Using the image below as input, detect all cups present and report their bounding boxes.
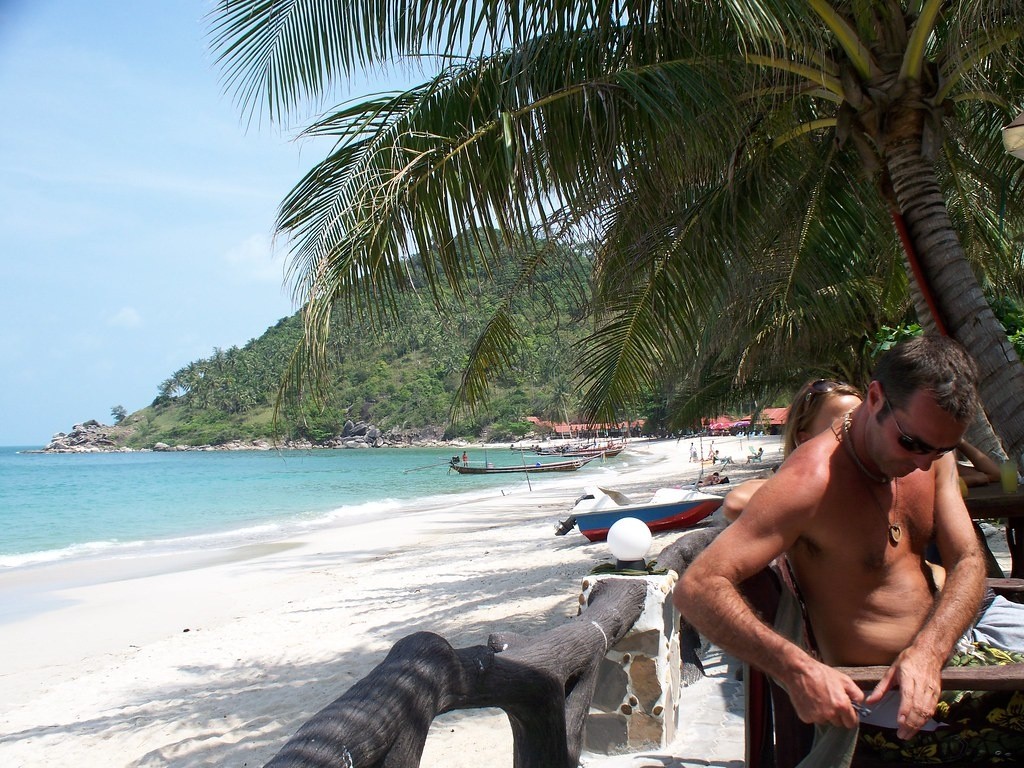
[999,460,1017,494]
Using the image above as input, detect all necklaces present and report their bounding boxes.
[842,402,892,484]
[831,413,901,543]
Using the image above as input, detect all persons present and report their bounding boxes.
[689,443,697,463]
[713,450,736,465]
[705,440,716,461]
[746,448,762,463]
[691,472,722,487]
[462,451,468,467]
[673,336,1024,768]
[531,439,615,453]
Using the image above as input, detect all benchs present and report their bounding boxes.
[743,554,1024,768]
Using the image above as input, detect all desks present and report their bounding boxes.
[964,481,1024,580]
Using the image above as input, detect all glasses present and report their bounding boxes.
[882,392,958,457]
[807,380,849,410]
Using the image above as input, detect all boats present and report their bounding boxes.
[537,441,625,458]
[552,487,730,543]
[447,451,606,474]
